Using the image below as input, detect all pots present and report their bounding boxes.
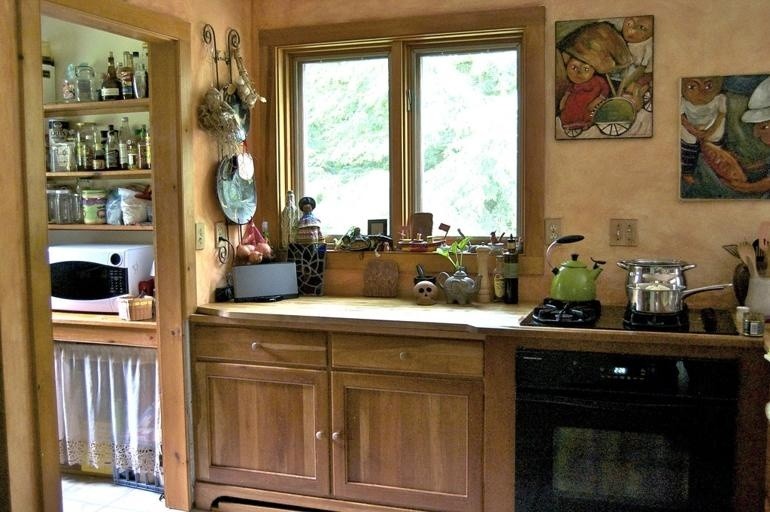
[626,277,732,310]
[616,255,697,290]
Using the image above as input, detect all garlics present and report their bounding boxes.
[239,242,272,263]
[234,76,259,107]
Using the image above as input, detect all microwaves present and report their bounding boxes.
[48,241,157,315]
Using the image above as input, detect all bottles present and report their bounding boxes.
[47,189,56,223]
[494,241,505,299]
[505,235,520,303]
[475,241,490,303]
[55,189,72,224]
[47,117,151,170]
[750,310,765,336]
[63,43,148,101]
[737,303,751,333]
[260,222,271,242]
[71,192,84,223]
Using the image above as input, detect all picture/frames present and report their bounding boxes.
[367,218,387,237]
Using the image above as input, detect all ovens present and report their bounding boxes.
[516,347,737,507]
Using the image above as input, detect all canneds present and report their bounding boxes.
[742,312,765,338]
[76,122,96,148]
[48,119,69,148]
[70,194,82,223]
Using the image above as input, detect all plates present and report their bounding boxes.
[218,84,251,142]
[216,153,257,225]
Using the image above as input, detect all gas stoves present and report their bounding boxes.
[518,298,739,336]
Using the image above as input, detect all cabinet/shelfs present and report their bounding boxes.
[189,316,485,511]
[43,95,151,232]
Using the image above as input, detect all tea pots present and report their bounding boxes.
[546,234,607,305]
[436,266,483,304]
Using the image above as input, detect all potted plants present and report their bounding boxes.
[435,237,482,306]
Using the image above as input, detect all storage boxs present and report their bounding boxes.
[42,64,56,105]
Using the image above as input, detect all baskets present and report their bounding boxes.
[119,296,153,321]
[110,436,164,494]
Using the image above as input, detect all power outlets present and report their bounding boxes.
[215,221,227,248]
[544,218,562,247]
